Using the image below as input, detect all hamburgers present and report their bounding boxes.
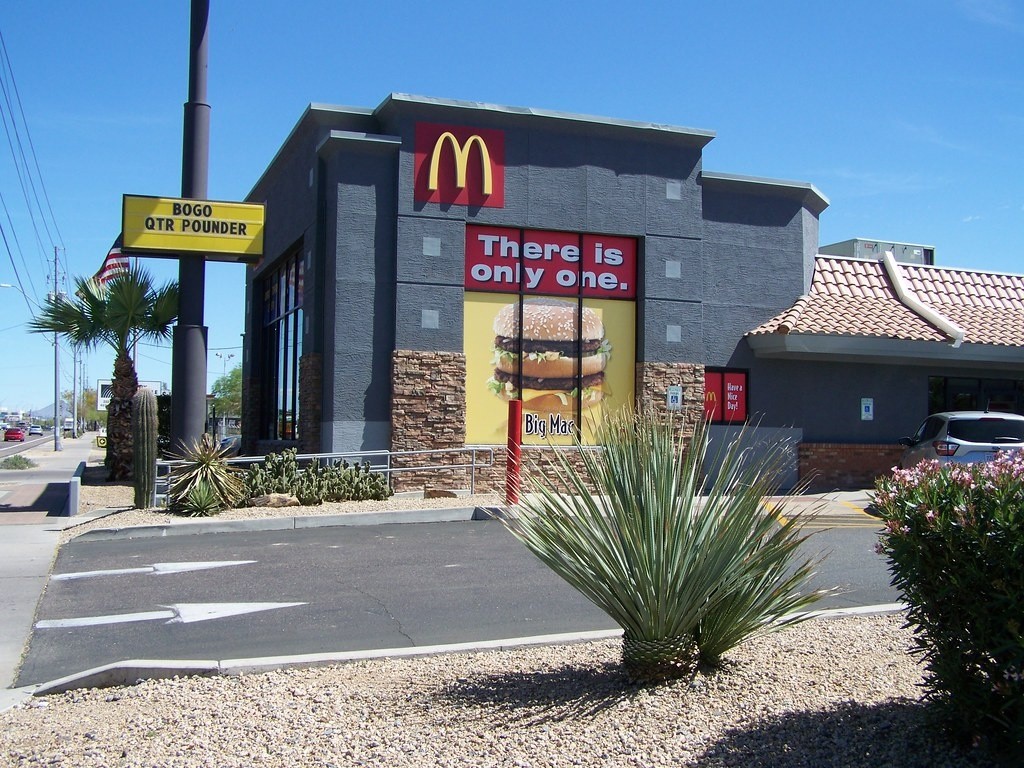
[486,297,612,411]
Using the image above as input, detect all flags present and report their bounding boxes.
[75,232,130,297]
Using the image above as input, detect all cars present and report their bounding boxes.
[4,427,24,442]
[898,408,1024,471]
[29,424,43,437]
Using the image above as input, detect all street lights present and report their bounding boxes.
[0,283,63,452]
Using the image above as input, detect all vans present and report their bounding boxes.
[63,417,74,432]
